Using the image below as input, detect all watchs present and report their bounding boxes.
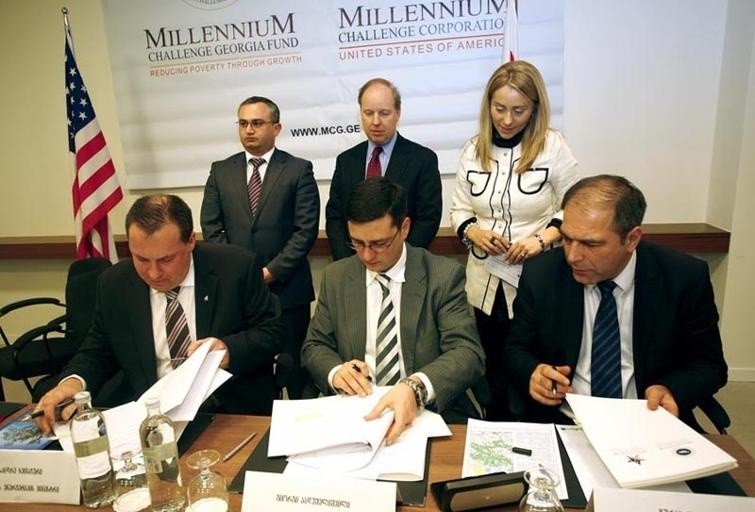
[400,377,427,417]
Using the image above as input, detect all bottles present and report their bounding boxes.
[139,398,187,511]
[69,392,117,508]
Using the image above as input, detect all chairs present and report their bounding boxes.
[1,258,112,404]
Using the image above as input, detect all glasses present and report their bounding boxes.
[236,118,279,127]
[344,218,406,254]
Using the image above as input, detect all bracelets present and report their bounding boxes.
[533,231,549,253]
[463,220,478,248]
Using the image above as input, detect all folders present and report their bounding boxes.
[43,409,215,472]
[541,423,749,508]
[226,421,432,506]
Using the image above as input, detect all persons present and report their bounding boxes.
[326,78,442,262]
[201,97,320,400]
[299,178,486,448]
[450,61,584,421]
[502,174,727,419]
[34,195,286,437]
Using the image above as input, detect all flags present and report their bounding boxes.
[62,6,123,264]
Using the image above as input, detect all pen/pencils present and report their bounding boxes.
[221,432,257,463]
[350,362,372,382]
[551,365,557,396]
[20,398,75,423]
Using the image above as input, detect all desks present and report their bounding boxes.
[0,395,755,511]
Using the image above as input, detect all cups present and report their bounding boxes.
[186,448,230,512]
[109,441,151,512]
[519,467,563,512]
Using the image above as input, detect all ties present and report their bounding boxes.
[365,145,385,181]
[245,156,267,223]
[590,278,624,400]
[164,285,194,369]
[374,273,401,388]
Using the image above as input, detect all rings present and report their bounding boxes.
[490,236,497,245]
